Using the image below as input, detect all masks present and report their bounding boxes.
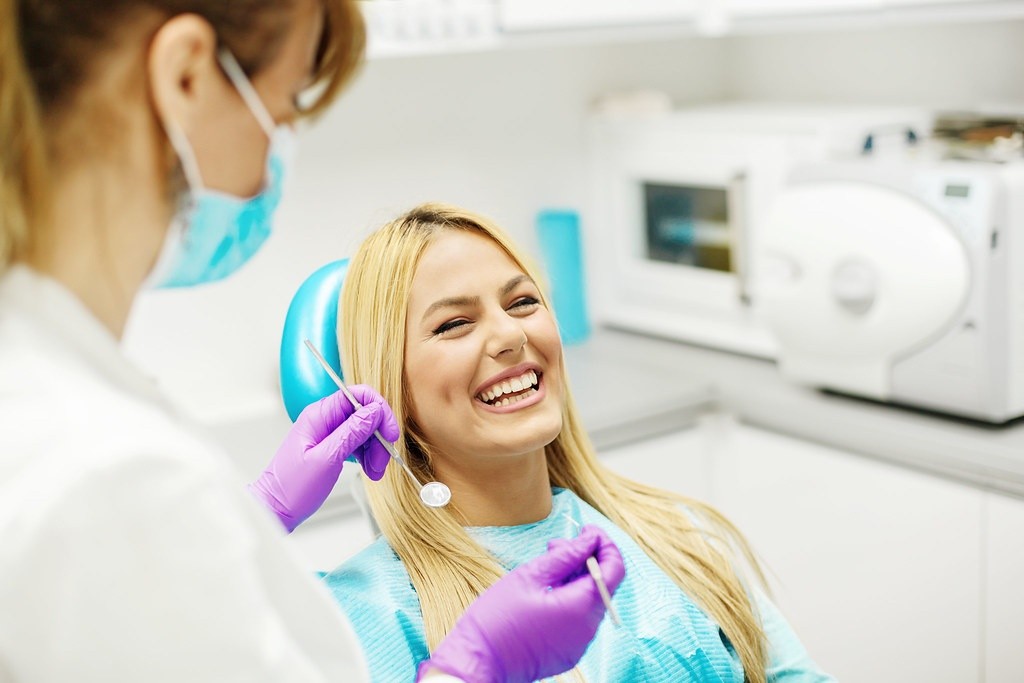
[146,46,299,290]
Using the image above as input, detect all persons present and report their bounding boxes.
[0,0,625,683]
[321,204,836,683]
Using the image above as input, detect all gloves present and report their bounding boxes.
[249,383,400,534]
[416,524,626,683]
